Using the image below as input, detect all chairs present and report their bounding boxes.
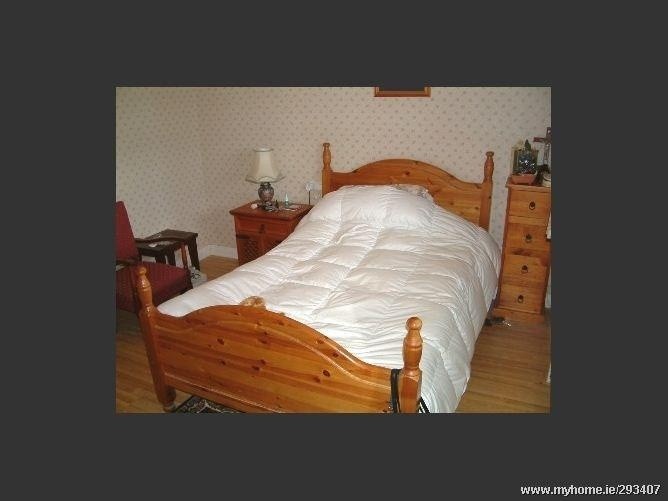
[115,201,193,313]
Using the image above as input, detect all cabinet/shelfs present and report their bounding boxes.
[491,176,552,325]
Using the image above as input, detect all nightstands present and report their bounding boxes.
[229,199,314,266]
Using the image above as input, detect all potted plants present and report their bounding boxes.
[511,139,537,185]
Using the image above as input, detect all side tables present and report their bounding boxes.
[139,228,200,272]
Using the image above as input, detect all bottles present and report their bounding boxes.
[284,193,289,208]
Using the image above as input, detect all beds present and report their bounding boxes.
[133,142,505,414]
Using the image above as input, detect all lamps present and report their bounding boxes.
[245,147,287,207]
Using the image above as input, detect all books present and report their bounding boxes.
[285,204,304,212]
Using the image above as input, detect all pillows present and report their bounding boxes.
[309,185,435,230]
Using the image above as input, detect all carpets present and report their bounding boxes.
[173,393,243,413]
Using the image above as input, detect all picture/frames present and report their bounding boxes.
[375,86,430,97]
